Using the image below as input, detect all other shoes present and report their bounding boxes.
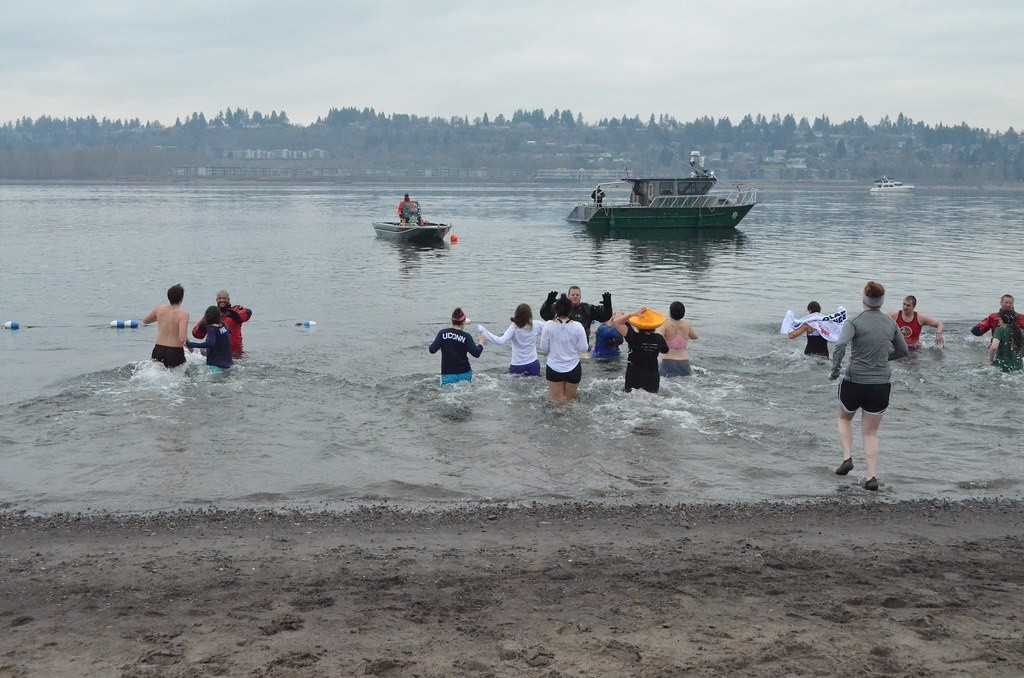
[863,476,878,490]
[836,456,853,475]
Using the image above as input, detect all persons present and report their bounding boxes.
[786,301,847,360]
[591,311,624,359]
[658,301,698,378]
[540,294,589,402]
[429,307,490,387]
[885,295,945,347]
[541,286,612,351]
[970,294,1024,349]
[827,281,907,491]
[143,283,194,369]
[183,306,233,368]
[591,184,605,207]
[478,303,544,377]
[192,289,252,358]
[614,307,669,393]
[398,192,411,223]
[990,309,1024,373]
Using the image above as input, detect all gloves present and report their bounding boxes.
[599,292,611,307]
[547,291,559,303]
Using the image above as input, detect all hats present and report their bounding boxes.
[628,308,665,329]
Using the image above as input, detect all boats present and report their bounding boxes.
[565,150,761,229]
[870,175,914,192]
[372,201,452,244]
